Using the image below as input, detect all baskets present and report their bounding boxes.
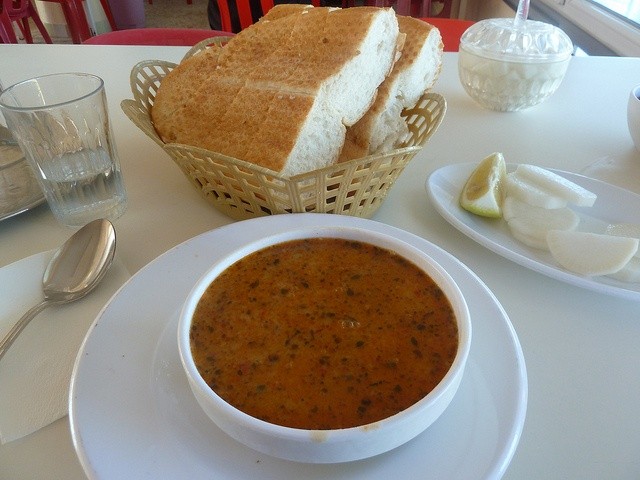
[121,35,447,222]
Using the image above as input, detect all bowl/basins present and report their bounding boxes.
[626,86,639,149]
[177,226,474,465]
[457,0,574,113]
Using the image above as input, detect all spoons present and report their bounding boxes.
[0,217,117,358]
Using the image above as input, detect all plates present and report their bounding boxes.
[424,159,640,305]
[67,212,530,480]
[1,136,46,221]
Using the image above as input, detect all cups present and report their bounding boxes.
[0,72,129,228]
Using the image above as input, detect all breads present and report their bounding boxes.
[151,19,404,209]
[334,19,444,185]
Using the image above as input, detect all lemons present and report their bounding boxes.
[458,150,508,219]
